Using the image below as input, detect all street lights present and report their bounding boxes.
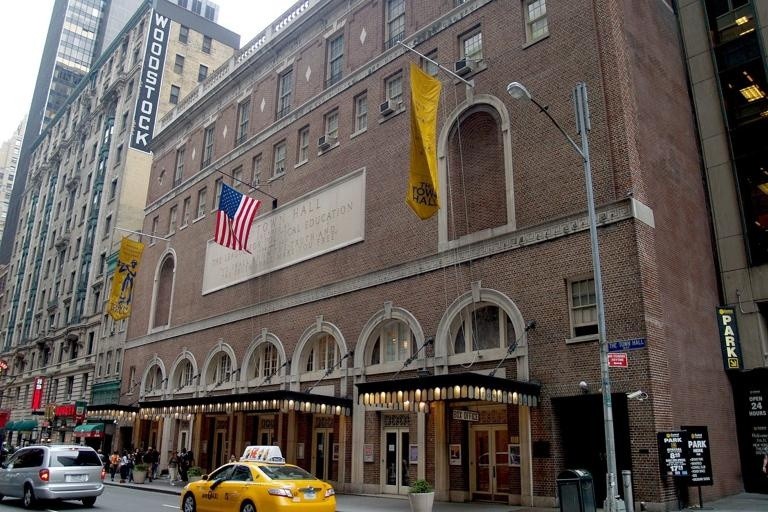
[506,82,635,512]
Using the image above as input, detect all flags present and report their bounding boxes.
[213,179,263,254]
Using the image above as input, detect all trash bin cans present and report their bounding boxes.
[556,469,596,512]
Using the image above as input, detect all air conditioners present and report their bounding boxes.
[454,57,472,76]
[379,100,395,116]
[318,135,330,150]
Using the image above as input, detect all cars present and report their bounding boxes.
[0,445,106,509]
[180,445,336,512]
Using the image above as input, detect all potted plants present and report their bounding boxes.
[408,479,435,512]
[186,466,206,483]
[132,462,149,484]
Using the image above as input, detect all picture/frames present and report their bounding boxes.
[296,442,305,460]
[363,444,374,463]
[409,444,418,465]
[449,444,461,466]
[508,444,521,467]
[332,443,339,461]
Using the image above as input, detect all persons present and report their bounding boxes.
[97,445,237,486]
